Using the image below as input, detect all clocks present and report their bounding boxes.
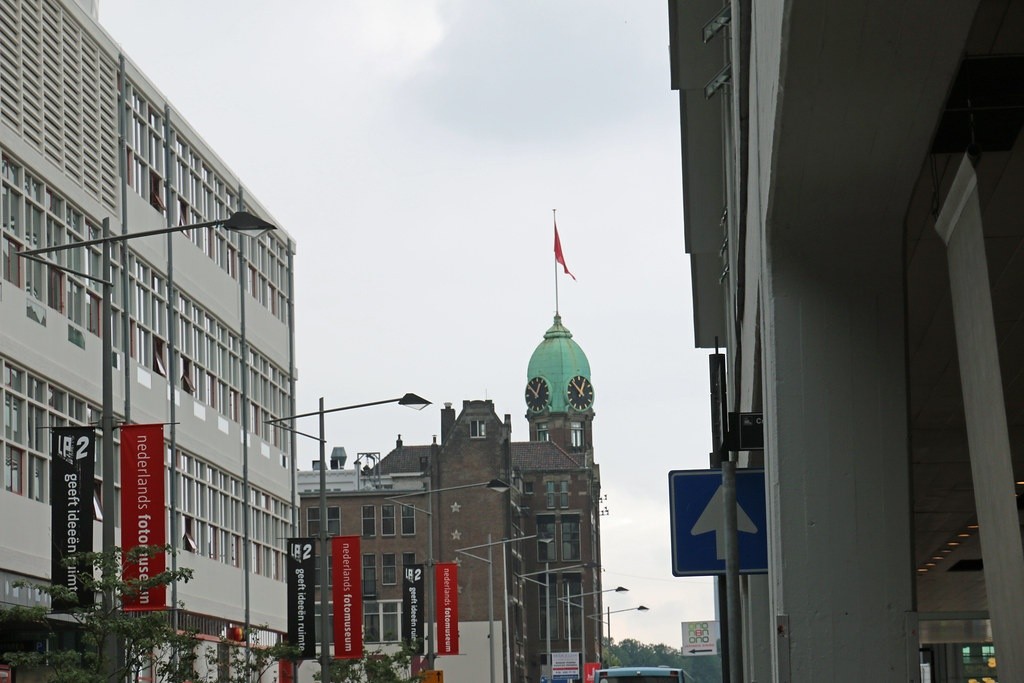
[524,375,553,414]
[563,374,594,413]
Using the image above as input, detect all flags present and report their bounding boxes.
[554,219,577,285]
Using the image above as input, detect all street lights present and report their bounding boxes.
[454,530,555,683]
[17,210,278,683]
[587,605,649,683]
[384,479,511,683]
[557,585,629,683]
[514,560,601,683]
[263,393,433,683]
